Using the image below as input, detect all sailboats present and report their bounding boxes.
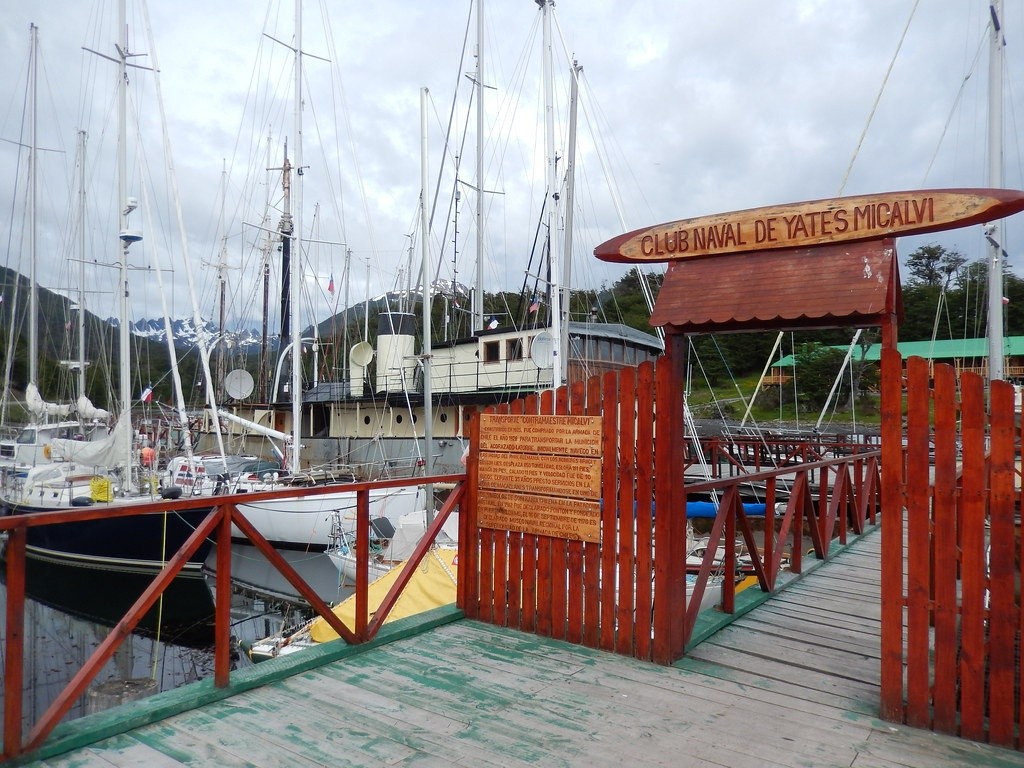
[1,0,789,660]
[882,0,1024,588]
